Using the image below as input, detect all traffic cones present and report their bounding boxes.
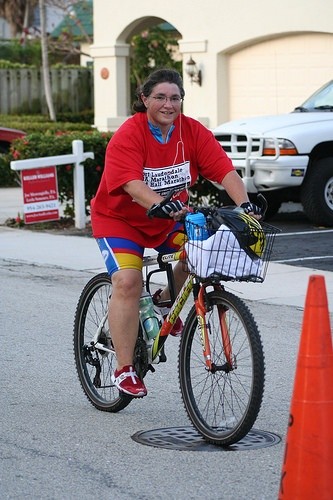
[277,273,333,500]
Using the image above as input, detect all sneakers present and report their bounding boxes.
[151,288,183,336]
[110,364,148,399]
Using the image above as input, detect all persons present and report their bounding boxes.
[90,69,263,397]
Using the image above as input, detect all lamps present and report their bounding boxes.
[186,56,203,87]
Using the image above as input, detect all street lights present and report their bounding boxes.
[183,55,202,87]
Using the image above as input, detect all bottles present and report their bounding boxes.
[185,207,210,241]
[139,287,159,340]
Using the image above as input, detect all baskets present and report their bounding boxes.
[181,214,283,283]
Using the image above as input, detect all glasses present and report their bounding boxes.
[144,93,184,105]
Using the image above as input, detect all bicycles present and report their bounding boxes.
[72,187,283,448]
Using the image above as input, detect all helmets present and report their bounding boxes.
[212,210,266,259]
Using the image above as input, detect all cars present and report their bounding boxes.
[0,125,28,155]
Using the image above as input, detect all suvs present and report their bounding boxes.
[206,76,333,230]
